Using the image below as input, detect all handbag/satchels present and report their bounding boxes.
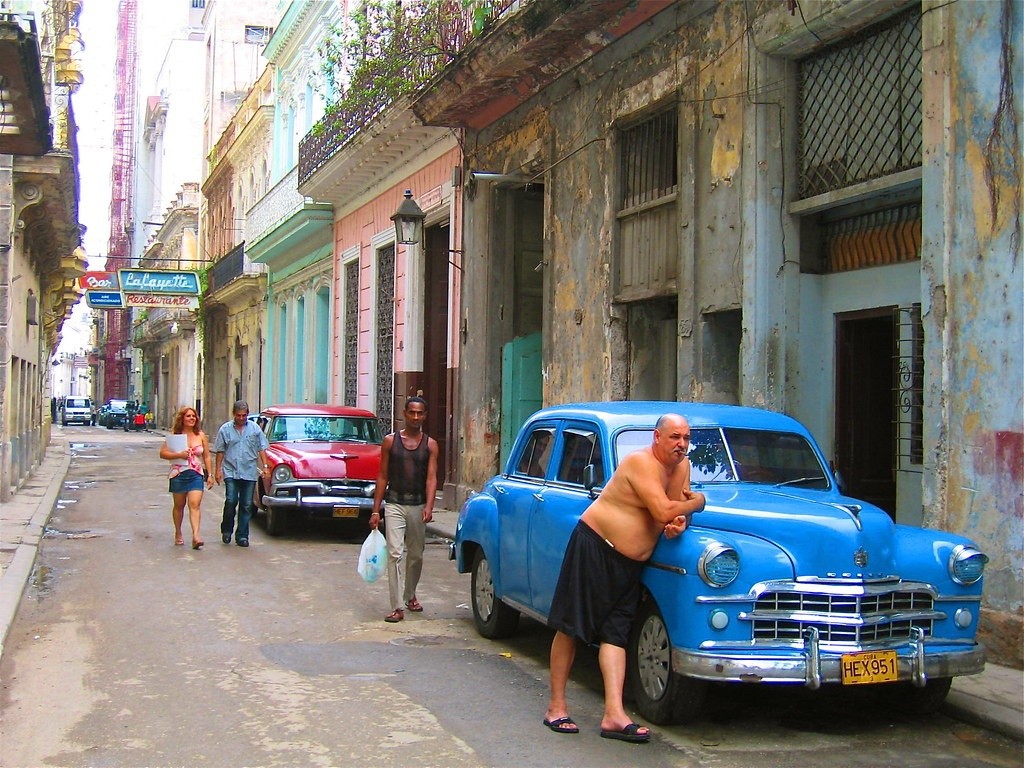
[357,527,388,584]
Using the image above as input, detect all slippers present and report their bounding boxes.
[543,717,579,733]
[192,541,205,549]
[384,610,404,622]
[174,532,184,545]
[600,724,651,742]
[404,596,423,612]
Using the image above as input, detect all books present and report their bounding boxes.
[166,434,188,466]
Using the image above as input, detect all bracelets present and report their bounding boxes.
[208,473,214,478]
[372,513,380,515]
[263,463,270,468]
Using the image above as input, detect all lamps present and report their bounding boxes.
[388,190,466,276]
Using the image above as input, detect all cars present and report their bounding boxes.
[254,405,387,536]
[448,399,992,726]
[99,399,136,429]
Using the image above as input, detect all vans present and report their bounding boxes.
[61,396,94,426]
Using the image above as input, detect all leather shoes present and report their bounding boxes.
[222,535,232,544]
[237,540,249,547]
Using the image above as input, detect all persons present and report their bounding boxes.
[143,409,154,430]
[160,407,214,549]
[215,400,271,547]
[542,413,706,743]
[90,402,97,426]
[133,410,147,432]
[369,397,439,623]
